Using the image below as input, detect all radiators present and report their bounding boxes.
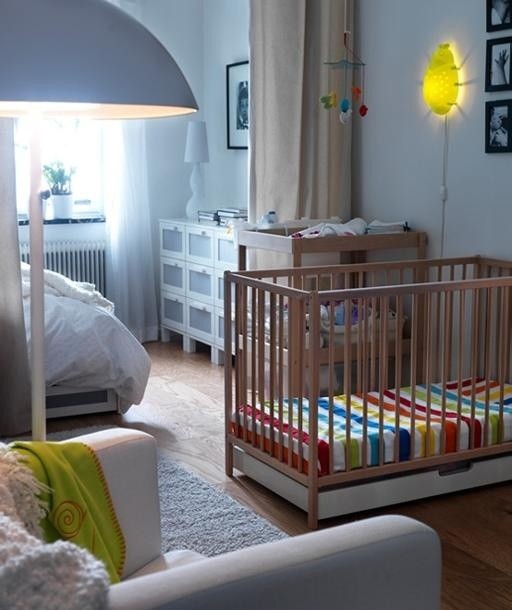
[19,238,112,308]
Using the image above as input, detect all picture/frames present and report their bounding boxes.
[484,99,511,152]
[485,38,511,92]
[225,60,251,149]
[486,0,512,32]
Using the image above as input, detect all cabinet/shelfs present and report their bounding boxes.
[229,223,428,393]
[157,218,237,363]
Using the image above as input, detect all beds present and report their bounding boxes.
[20,266,150,421]
[223,255,512,531]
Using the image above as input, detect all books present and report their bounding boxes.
[196,207,246,227]
[365,219,408,235]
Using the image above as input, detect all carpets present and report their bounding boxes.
[154,456,287,554]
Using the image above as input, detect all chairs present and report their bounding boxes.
[1,427,443,610]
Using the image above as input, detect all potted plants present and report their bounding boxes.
[43,162,76,221]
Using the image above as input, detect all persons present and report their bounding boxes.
[493,48,509,86]
[236,86,248,128]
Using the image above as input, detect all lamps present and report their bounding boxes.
[0,0,198,444]
[184,123,209,221]
[421,42,461,116]
